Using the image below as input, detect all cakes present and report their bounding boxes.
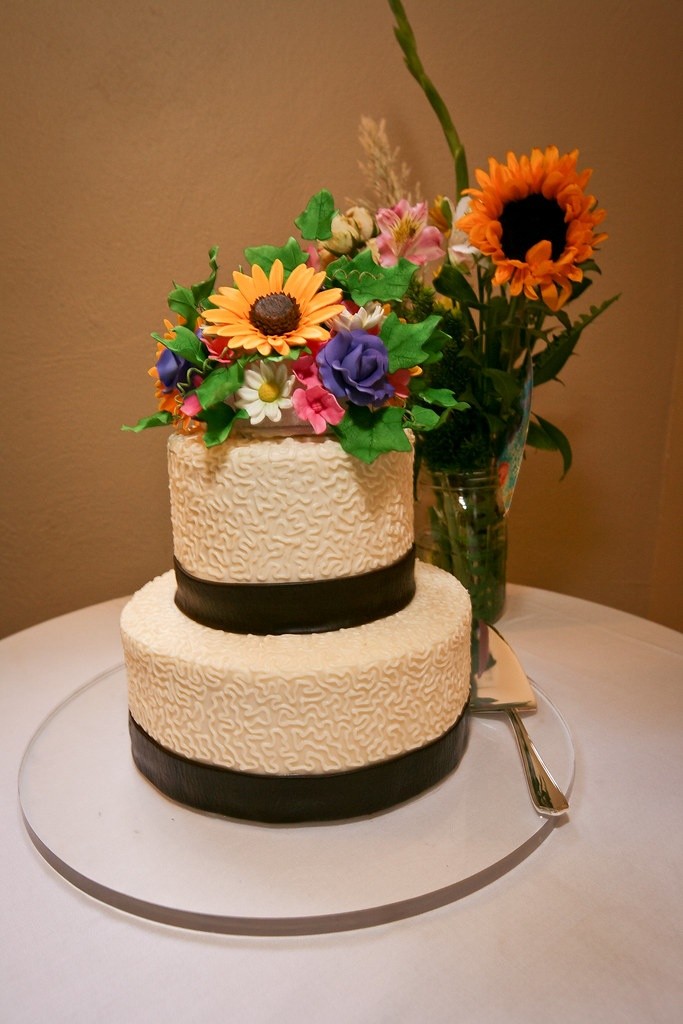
[121,233,471,824]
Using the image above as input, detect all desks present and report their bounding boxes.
[0,582,683,1024]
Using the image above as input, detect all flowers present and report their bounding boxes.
[119,0,617,483]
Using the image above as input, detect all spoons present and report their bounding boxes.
[467,619,571,816]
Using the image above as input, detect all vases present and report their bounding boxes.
[235,360,333,438]
[418,361,539,625]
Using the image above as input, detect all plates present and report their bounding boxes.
[17,658,574,932]
